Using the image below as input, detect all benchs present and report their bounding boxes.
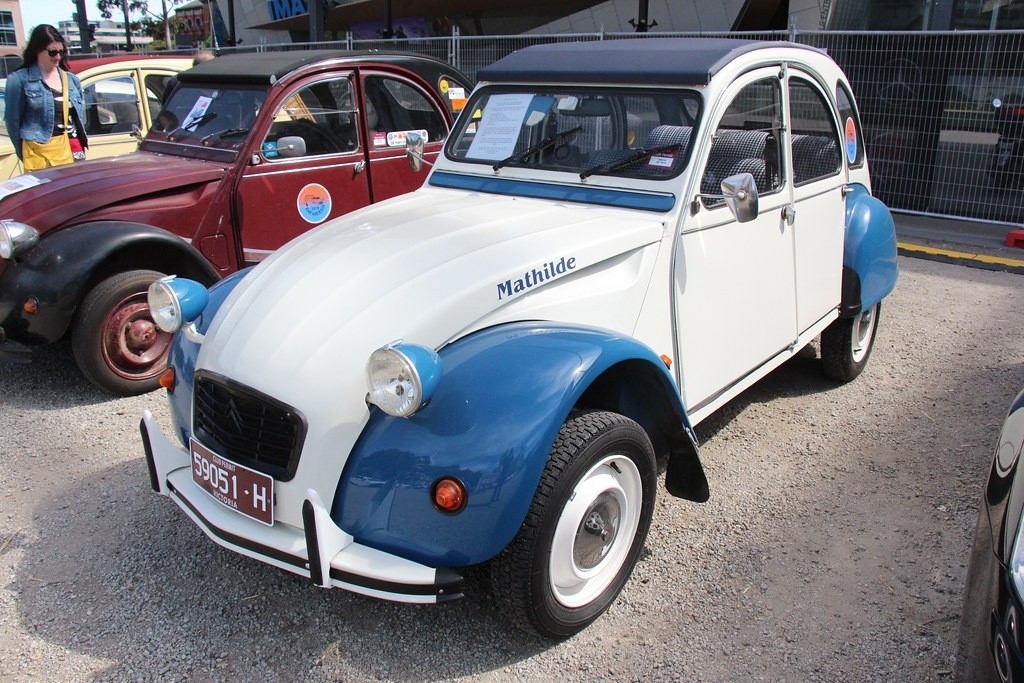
[582,124,838,195]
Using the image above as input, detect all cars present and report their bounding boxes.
[0,56,316,180]
[952,389,1024,683]
[0,48,487,395]
[138,32,897,641]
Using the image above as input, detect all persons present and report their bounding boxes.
[178,51,242,140]
[4,24,90,170]
[154,109,178,132]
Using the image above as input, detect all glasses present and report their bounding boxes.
[44,47,66,58]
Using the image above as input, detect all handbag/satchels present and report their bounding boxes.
[20,132,75,173]
